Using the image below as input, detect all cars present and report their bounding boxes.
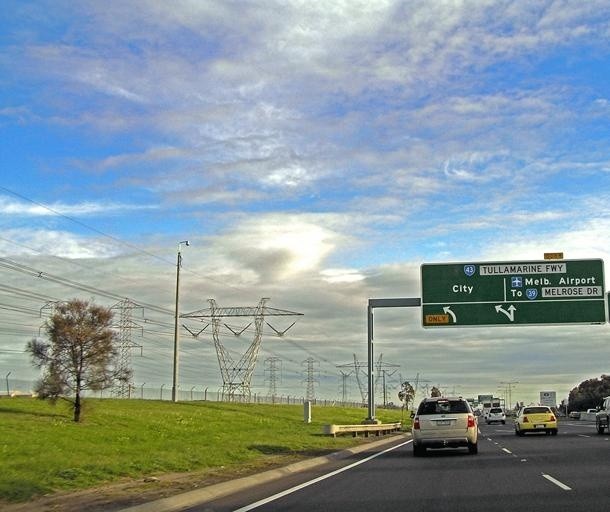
[587,408,598,413]
[512,405,558,437]
[473,412,479,426]
[595,394,610,433]
[506,409,517,417]
[557,411,566,418]
[569,411,579,418]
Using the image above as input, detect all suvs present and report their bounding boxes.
[409,394,480,455]
[486,407,506,424]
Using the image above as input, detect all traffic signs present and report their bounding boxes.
[420,259,609,328]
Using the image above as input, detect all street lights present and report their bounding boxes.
[169,238,190,401]
[497,381,520,409]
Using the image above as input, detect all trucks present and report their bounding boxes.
[482,400,501,419]
